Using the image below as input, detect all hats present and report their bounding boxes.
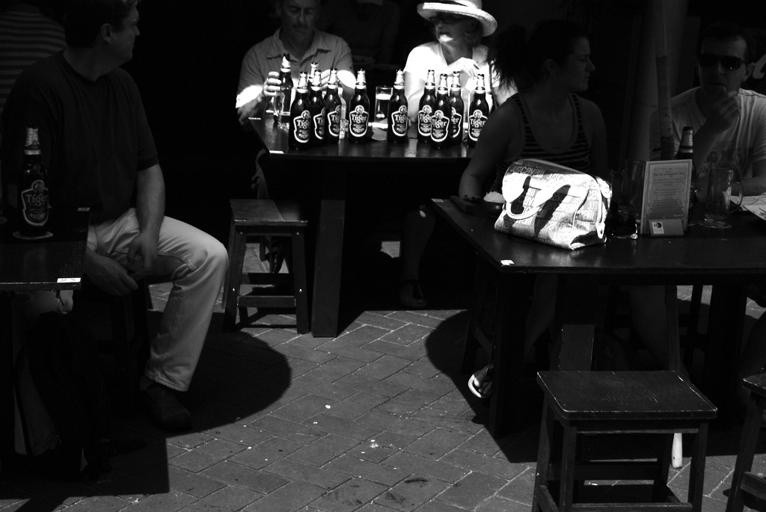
[416,0,498,37]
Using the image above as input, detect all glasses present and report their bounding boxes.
[698,51,746,71]
[428,16,462,25]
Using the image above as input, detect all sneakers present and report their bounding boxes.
[399,281,428,309]
[142,380,185,422]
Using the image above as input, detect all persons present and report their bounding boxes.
[317,1,400,63]
[2,7,57,90]
[457,19,611,399]
[1,1,231,485]
[394,0,519,310]
[627,24,766,433]
[236,1,365,282]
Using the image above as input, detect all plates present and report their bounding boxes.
[13,231,54,240]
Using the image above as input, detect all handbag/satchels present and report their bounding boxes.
[494,158,613,250]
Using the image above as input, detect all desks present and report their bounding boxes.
[247,114,475,337]
[427,196,766,438]
[2,205,93,480]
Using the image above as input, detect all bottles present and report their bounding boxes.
[673,125,695,219]
[273,52,489,150]
[16,122,50,236]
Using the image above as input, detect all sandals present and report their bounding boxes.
[467,363,496,401]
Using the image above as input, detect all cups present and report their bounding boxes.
[704,166,744,229]
[614,158,646,225]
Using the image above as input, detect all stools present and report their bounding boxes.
[98,280,151,422]
[222,197,309,333]
[533,370,717,511]
[727,373,766,512]
